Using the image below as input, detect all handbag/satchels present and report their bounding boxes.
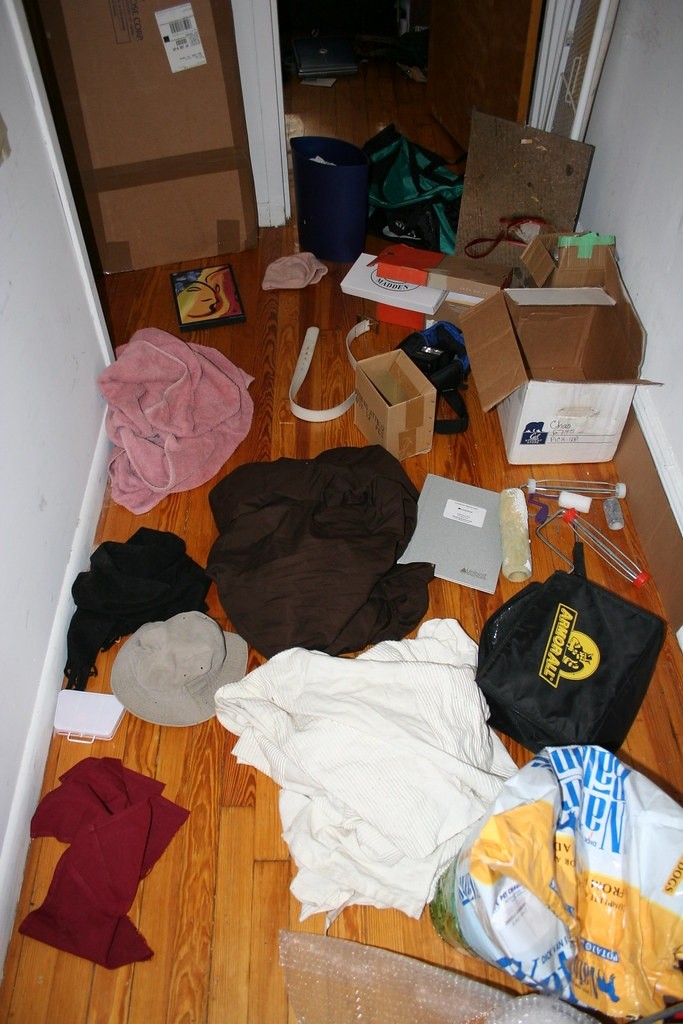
[362,123,465,257]
[395,321,472,436]
[475,570,662,754]
[465,212,559,260]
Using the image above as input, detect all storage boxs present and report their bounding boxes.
[170,267,247,333]
[340,229,665,465]
[36,0,259,277]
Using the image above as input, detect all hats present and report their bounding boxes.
[109,610,248,728]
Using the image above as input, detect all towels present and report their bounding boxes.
[96,328,256,516]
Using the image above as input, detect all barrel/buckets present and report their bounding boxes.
[290,136,372,263]
